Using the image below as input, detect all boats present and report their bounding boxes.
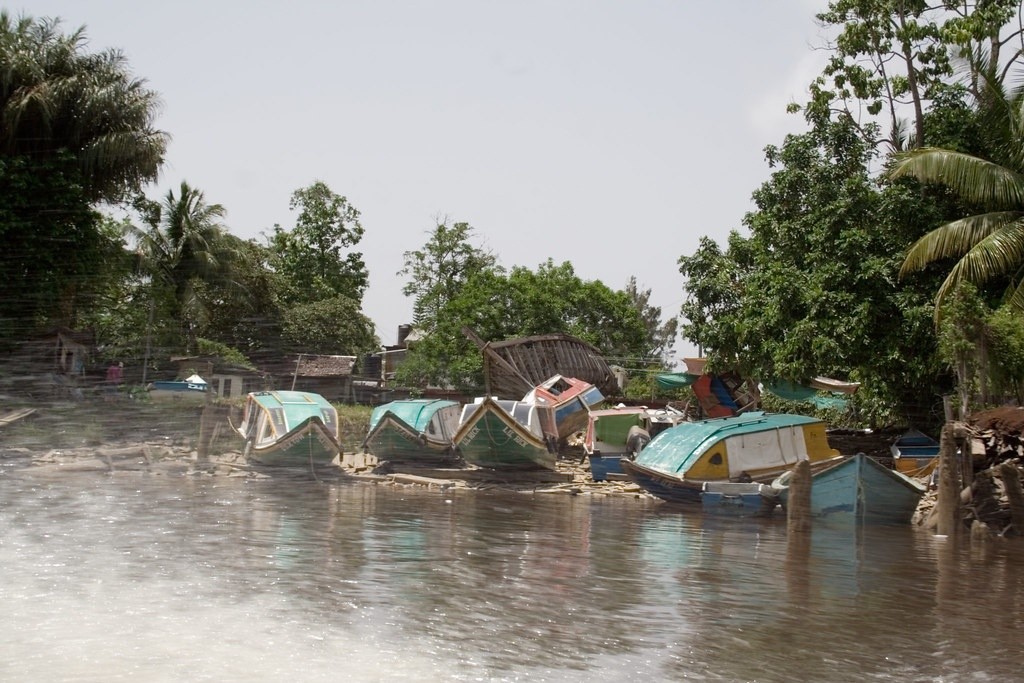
[226,388,342,467]
[452,394,561,472]
[458,325,622,405]
[521,374,607,444]
[579,355,939,526]
[146,372,217,407]
[359,397,462,464]
